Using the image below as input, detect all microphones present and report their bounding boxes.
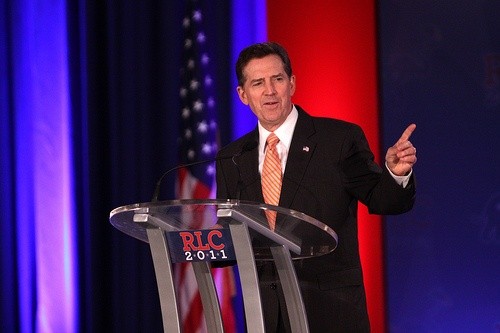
[153,145,250,201]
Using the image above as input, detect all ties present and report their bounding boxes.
[261,133,283,231]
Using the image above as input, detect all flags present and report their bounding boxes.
[172,1,237,333]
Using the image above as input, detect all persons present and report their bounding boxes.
[217,41,417,332]
